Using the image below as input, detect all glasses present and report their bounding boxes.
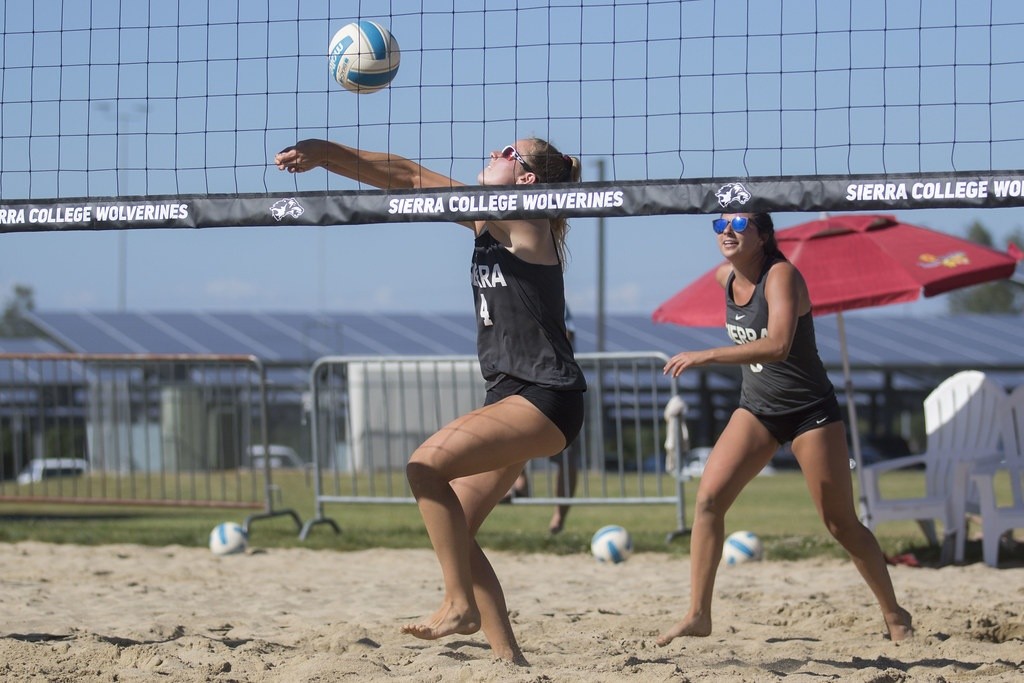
[502,145,539,184]
[712,216,765,235]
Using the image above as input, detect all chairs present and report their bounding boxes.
[965,383,1024,567]
[859,371,1009,562]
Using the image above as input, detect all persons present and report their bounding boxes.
[506,303,580,534]
[274,139,579,665]
[653,213,913,647]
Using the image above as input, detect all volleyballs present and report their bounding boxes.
[589,524,633,563]
[326,18,402,96]
[721,531,764,568]
[208,521,247,556]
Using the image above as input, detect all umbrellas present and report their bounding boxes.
[652,212,1016,518]
